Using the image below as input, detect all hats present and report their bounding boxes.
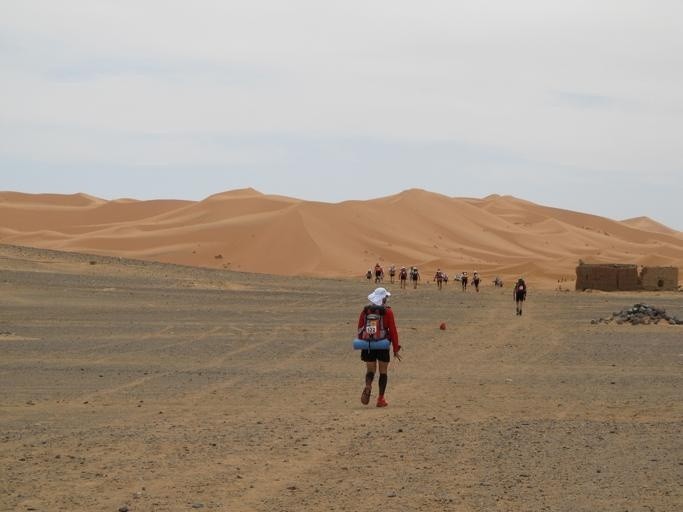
[369,287,391,299]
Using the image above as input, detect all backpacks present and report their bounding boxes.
[515,280,525,293]
[367,267,480,281]
[359,305,389,341]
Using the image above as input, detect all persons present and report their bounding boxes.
[356,286,405,408]
[511,277,527,317]
[363,261,482,294]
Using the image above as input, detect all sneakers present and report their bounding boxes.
[360,385,371,405]
[376,399,388,407]
